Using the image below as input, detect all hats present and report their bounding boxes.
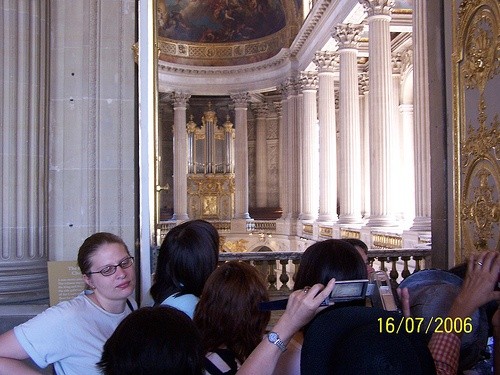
[300,306,437,375]
[397,270,491,369]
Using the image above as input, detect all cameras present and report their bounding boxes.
[325,271,400,312]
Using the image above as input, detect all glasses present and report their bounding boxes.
[86,257,135,277]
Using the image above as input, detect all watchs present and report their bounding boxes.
[267,331,287,352]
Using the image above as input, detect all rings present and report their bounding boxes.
[303,286,309,293]
[474,261,483,266]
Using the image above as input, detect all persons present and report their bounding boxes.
[0,232,138,375]
[428,249,500,375]
[265,239,368,375]
[397,270,489,370]
[193,260,271,375]
[339,238,375,307]
[446,263,498,375]
[233,278,436,375]
[140,218,219,319]
[96,302,205,375]
